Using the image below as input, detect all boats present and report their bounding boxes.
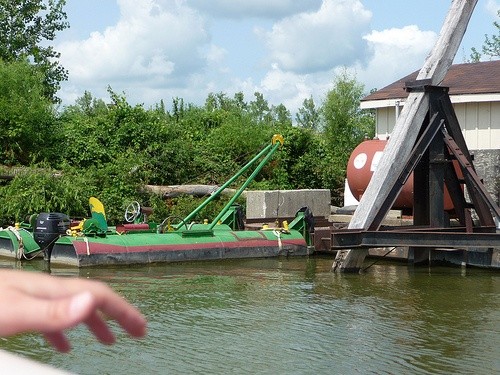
[0,204,160,260]
[47,135,305,266]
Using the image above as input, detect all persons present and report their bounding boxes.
[0,266,147,374]
